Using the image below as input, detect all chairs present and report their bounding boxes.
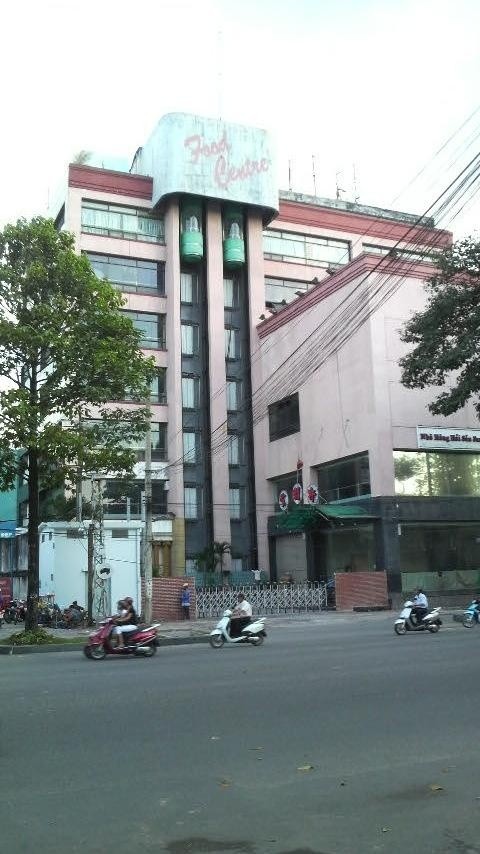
[118,643,124,648]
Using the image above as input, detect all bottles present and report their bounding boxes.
[183,583,188,587]
[237,594,244,598]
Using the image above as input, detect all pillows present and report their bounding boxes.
[118,597,131,610]
[415,587,422,592]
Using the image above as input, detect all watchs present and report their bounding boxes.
[393,599,443,635]
[463,599,480,629]
[85,615,162,661]
[209,609,268,648]
[3,597,26,625]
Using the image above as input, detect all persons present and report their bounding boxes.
[117,602,130,617]
[110,597,138,649]
[54,601,86,628]
[230,592,253,636]
[411,586,428,624]
[181,583,192,620]
